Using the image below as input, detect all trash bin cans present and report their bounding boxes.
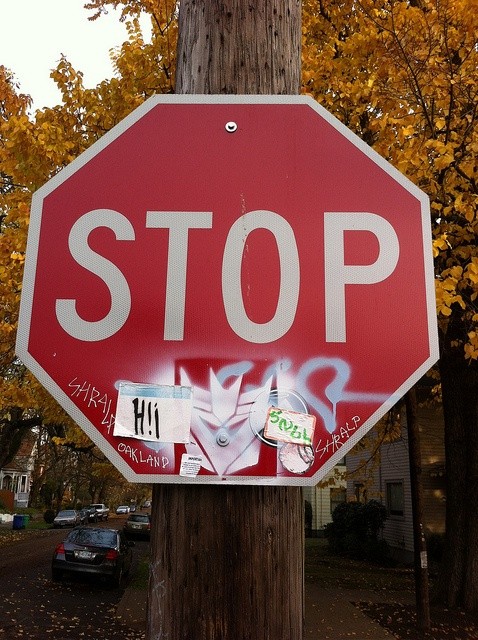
[12,515,23,530]
[23,515,30,530]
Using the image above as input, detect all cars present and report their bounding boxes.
[53,509,81,528]
[116,505,130,515]
[51,527,136,588]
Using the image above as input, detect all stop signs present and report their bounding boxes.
[10,95,442,487]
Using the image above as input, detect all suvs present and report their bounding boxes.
[122,513,150,539]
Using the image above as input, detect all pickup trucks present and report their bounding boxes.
[90,503,109,521]
